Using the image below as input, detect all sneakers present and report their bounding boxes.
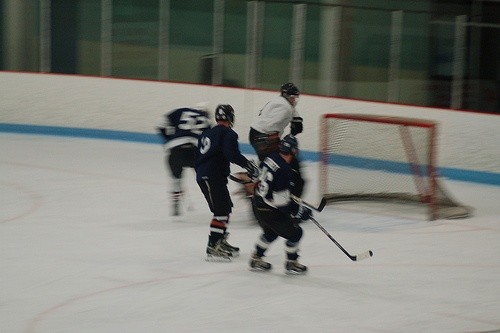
[248,253,272,272]
[205,234,233,262]
[283,256,307,276]
[221,232,240,257]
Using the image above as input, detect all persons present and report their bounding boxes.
[194,104,261,263]
[157,107,214,215]
[234,82,304,200]
[247,133,312,275]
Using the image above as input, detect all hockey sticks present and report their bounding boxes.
[309,214,374,263]
[292,194,329,214]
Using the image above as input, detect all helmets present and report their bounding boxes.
[194,103,209,112]
[215,104,234,122]
[280,82,301,98]
[277,134,297,155]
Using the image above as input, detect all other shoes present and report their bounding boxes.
[170,194,182,215]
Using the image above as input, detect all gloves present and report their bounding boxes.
[291,117,303,136]
[290,204,312,221]
[245,160,260,180]
